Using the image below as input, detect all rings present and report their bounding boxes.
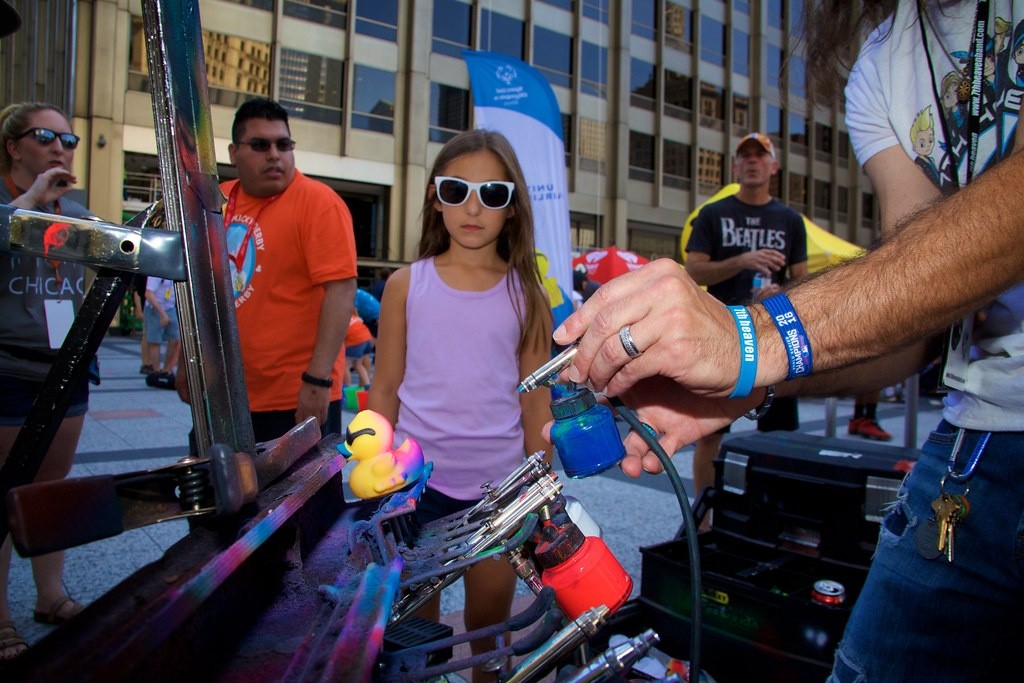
[619,324,641,358]
[628,422,657,440]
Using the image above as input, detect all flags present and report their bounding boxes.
[464,48,572,349]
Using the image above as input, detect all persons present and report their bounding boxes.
[572,271,600,304]
[175,98,358,563]
[345,289,380,386]
[367,130,555,683]
[684,133,810,532]
[131,273,182,375]
[0,103,100,660]
[847,392,894,441]
[542,0,1024,683]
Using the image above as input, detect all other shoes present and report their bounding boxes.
[140,365,163,374]
[885,392,907,402]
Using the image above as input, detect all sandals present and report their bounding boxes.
[33,594,86,625]
[0,620,30,662]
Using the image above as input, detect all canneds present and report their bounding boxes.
[811,580,846,605]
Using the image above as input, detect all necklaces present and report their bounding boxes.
[923,0,980,101]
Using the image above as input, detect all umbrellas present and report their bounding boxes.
[572,246,651,284]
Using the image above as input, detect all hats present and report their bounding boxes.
[735,133,774,159]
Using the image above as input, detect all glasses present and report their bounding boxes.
[435,175,515,210]
[12,127,80,150]
[233,137,296,152]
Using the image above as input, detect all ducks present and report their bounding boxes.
[337,410,425,499]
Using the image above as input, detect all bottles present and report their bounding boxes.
[752,269,771,306]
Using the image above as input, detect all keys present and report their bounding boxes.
[915,493,970,562]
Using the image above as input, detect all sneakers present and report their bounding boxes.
[848,416,892,442]
[146,372,177,391]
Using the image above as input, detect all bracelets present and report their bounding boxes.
[727,305,757,406]
[760,293,814,380]
[302,372,333,387]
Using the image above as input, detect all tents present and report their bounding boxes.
[680,181,869,291]
